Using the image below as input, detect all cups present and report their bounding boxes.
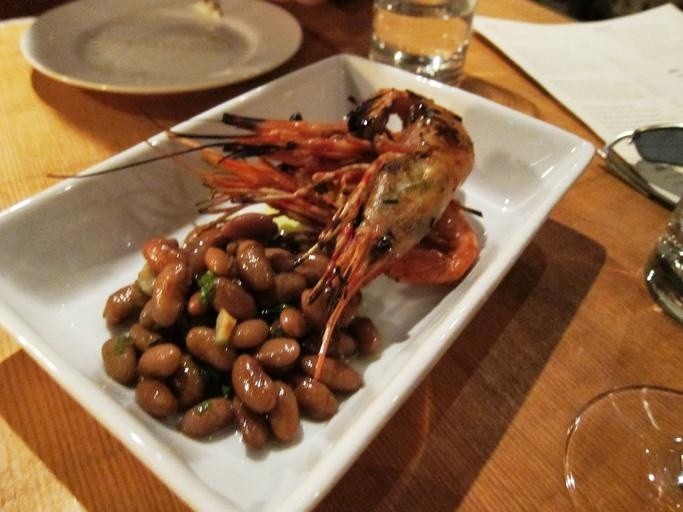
[367,0,475,89]
[643,191,683,327]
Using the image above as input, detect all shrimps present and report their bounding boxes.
[46,87,481,386]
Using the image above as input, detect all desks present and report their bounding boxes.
[0,4,683,512]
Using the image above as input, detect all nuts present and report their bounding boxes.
[101,212,379,448]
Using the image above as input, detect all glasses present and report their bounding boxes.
[598,125,683,197]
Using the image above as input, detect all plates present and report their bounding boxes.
[0,53,598,512]
[20,0,304,95]
